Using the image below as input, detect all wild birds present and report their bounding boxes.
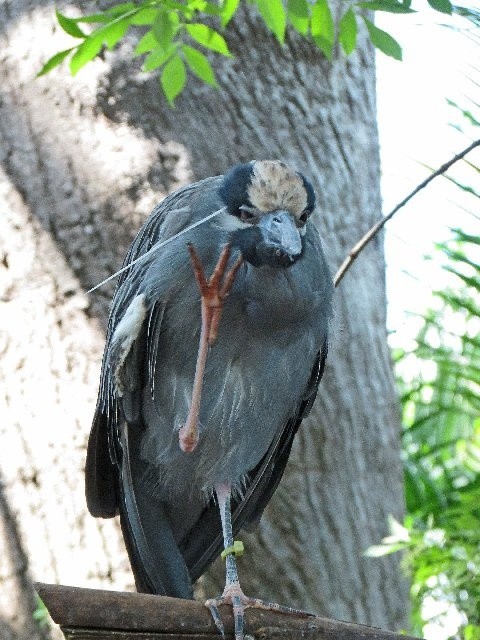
[83,156,335,640]
